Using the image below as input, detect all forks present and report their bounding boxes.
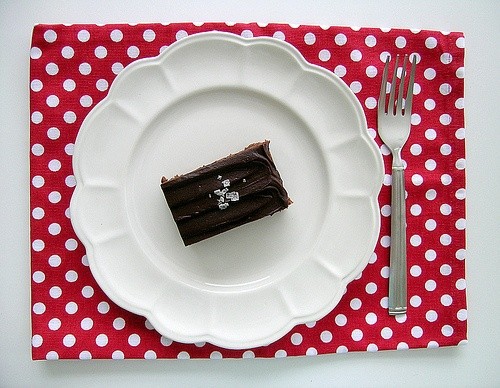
[377,54,417,315]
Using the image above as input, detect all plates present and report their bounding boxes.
[68,32,386,351]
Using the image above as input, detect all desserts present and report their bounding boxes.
[160,140,294,247]
